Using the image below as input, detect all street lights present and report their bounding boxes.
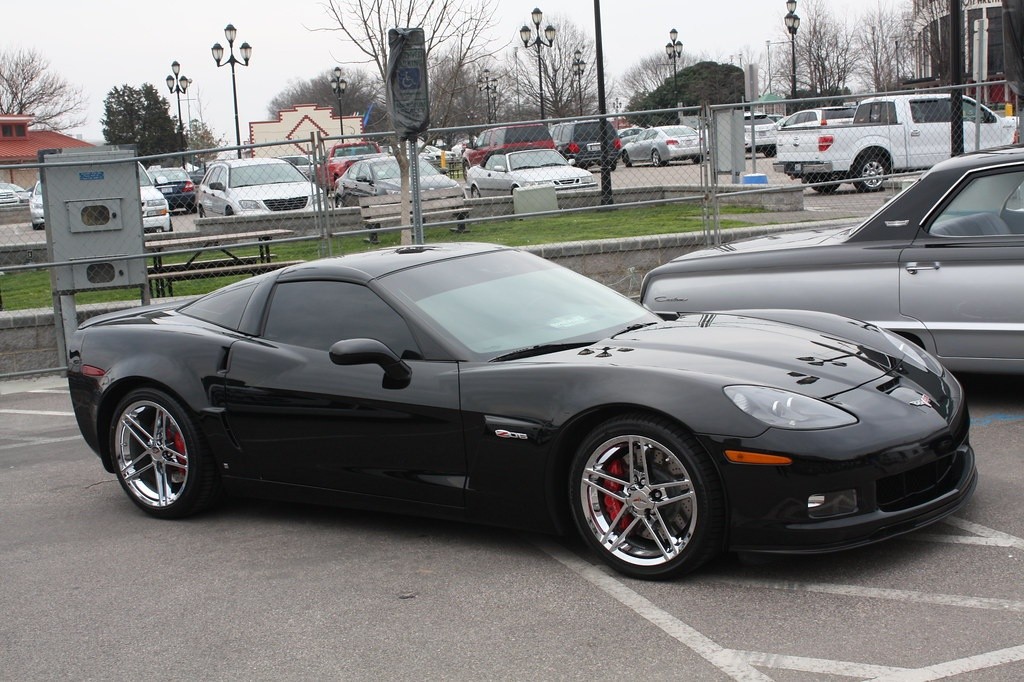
[766,40,773,94]
[212,23,252,158]
[572,50,586,116]
[166,61,190,168]
[784,0,799,113]
[871,26,877,92]
[520,7,556,118]
[666,27,683,106]
[330,66,346,144]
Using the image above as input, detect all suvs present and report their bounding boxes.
[549,120,621,171]
[463,124,557,181]
[698,111,778,157]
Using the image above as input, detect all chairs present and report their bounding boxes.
[275,285,415,349]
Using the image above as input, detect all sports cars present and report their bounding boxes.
[67,241,980,579]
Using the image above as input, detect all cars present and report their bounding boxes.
[616,124,708,167]
[139,160,207,232]
[197,158,323,218]
[386,136,477,159]
[638,145,1024,373]
[465,146,598,197]
[281,156,321,178]
[768,106,855,128]
[0,178,46,229]
[333,156,461,208]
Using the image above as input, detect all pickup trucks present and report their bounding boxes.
[772,94,1020,192]
[317,141,383,188]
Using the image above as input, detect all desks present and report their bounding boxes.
[145,227,293,299]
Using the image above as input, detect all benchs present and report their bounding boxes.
[934,212,1013,235]
[148,252,306,280]
[357,189,473,244]
